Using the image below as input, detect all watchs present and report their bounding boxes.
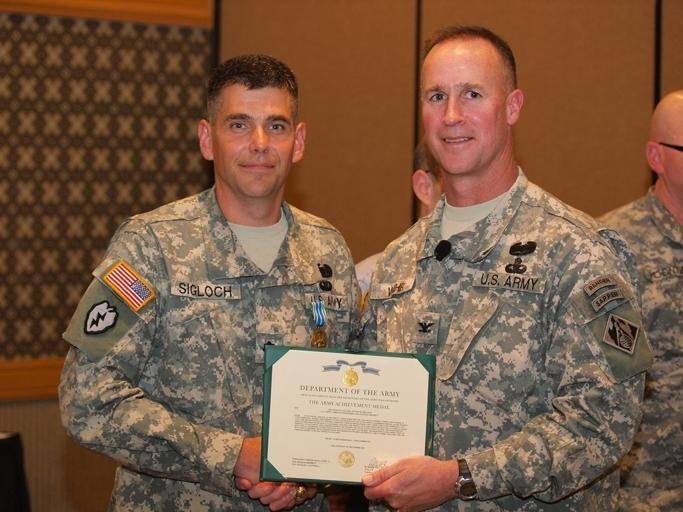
[453,458,478,501]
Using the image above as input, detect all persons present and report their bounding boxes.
[56,54,362,512]
[234,25,653,512]
[353,134,444,299]
[597,88,683,512]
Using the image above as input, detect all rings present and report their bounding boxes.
[293,485,308,505]
